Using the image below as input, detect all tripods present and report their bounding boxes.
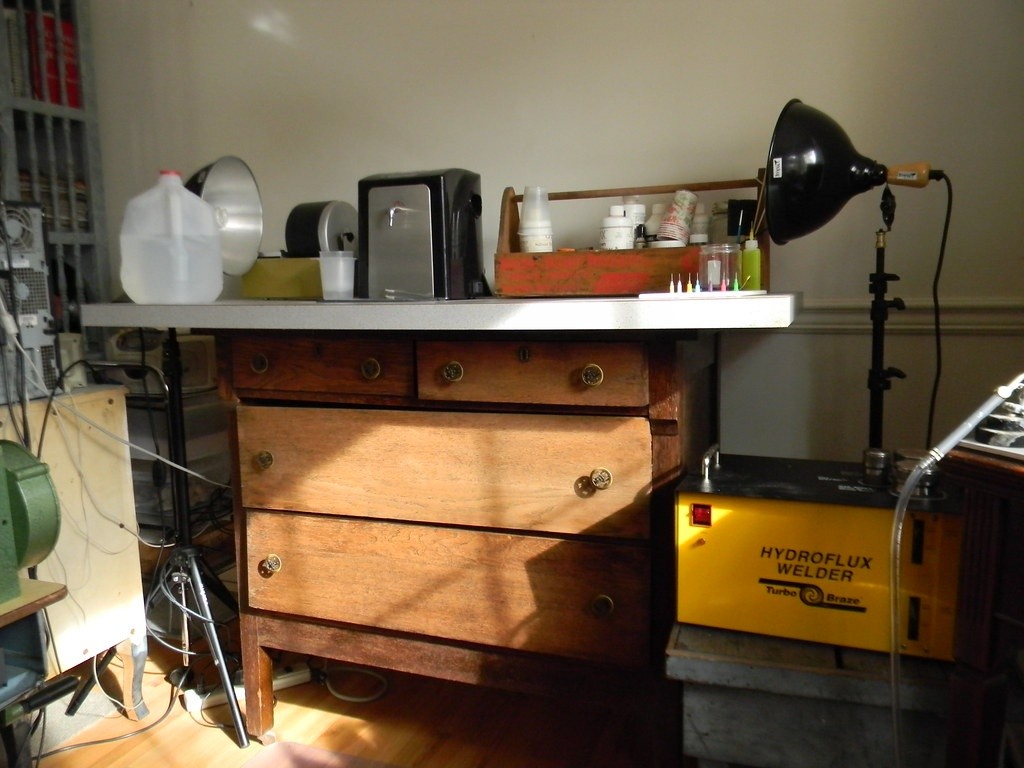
[66,331,252,750]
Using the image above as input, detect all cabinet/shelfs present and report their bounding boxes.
[75,292,798,768]
[0,0,110,363]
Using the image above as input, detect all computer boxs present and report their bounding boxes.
[0,198,65,405]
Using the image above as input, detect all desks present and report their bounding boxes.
[0,381,149,723]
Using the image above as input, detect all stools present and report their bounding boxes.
[662,622,953,768]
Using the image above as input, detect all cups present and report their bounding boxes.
[656,189,698,245]
[318,249,356,303]
[517,184,556,252]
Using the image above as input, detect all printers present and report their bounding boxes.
[105,328,218,395]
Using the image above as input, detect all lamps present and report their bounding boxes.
[138,154,267,749]
[764,96,954,448]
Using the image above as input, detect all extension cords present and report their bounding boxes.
[183,662,312,711]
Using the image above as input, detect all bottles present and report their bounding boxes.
[600,195,664,250]
[118,169,224,307]
[697,240,761,290]
[688,202,756,243]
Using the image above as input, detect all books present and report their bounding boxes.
[3,9,80,109]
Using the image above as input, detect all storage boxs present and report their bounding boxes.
[124,391,237,533]
[240,257,359,302]
[494,166,770,298]
